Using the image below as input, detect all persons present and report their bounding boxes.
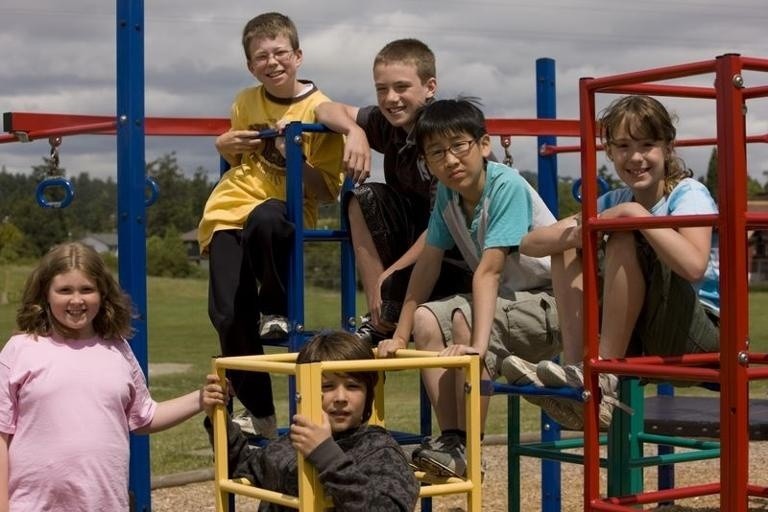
[200,330,419,512]
[196,11,348,439]
[314,39,496,350]
[377,96,563,484]
[0,241,236,512]
[502,95,720,430]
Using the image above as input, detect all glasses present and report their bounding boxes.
[421,137,478,162]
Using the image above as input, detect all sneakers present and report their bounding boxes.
[350,314,397,341]
[502,355,581,433]
[257,313,289,339]
[534,355,620,426]
[396,432,486,486]
[233,412,278,437]
